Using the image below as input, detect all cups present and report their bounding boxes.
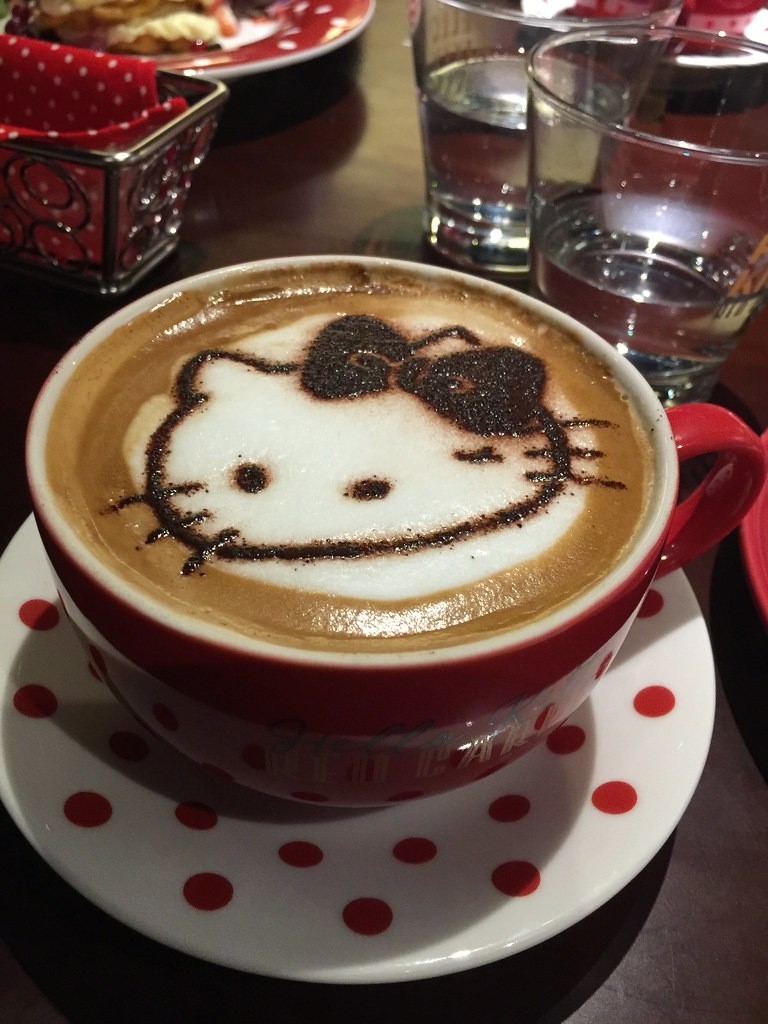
[407,0,682,274]
[577,0,768,53]
[21,254,768,811]
[526,26,768,410]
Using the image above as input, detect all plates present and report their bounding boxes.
[736,430,768,617]
[0,512,716,987]
[508,0,768,69]
[436,0,768,69]
[0,0,376,81]
